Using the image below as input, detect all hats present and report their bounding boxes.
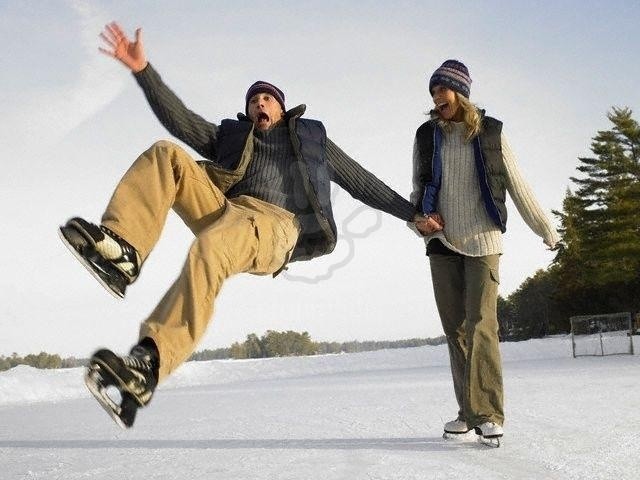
[429,59,472,98]
[245,80,286,116]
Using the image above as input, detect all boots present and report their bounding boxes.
[67,217,143,285]
[90,342,158,409]
[443,419,504,439]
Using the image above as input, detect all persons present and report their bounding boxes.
[67,20,443,405]
[406,61,556,438]
